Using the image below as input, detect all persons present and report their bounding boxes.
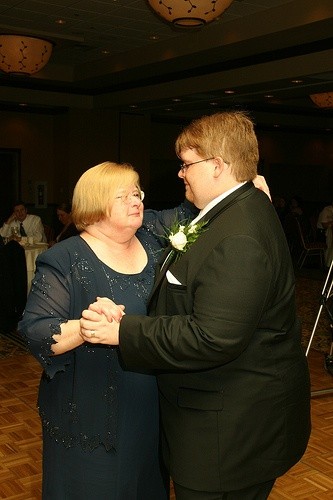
[16,161,273,500]
[272,195,306,241]
[316,205,333,244]
[0,201,48,246]
[48,201,79,248]
[79,111,312,500]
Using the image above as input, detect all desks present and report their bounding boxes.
[24,242,48,299]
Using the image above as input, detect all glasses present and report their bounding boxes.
[180,157,230,173]
[113,190,145,203]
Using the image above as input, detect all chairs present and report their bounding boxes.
[295,216,326,272]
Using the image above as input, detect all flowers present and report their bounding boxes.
[155,215,208,273]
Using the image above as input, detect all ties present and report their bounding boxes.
[19,223,27,237]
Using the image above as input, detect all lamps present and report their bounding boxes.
[309,92,333,109]
[148,0,234,31]
[0,34,57,78]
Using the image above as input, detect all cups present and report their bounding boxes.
[21,237,27,243]
[29,237,33,245]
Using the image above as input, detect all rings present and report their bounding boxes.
[91,330,95,338]
